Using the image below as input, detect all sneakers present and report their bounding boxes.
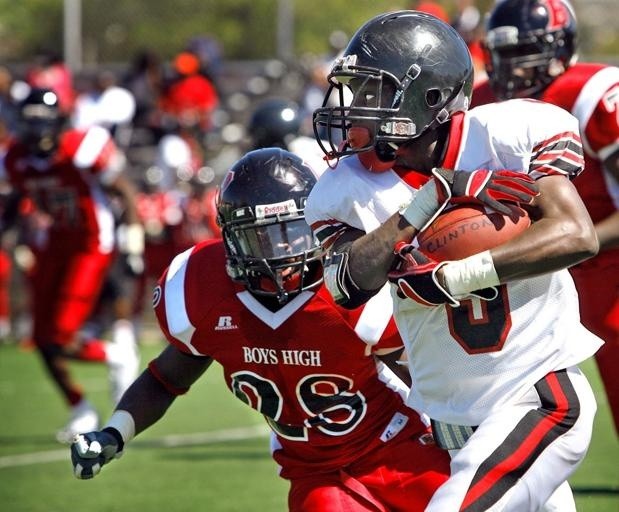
[105,321,139,403]
[60,409,96,445]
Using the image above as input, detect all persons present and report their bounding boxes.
[470,0,619,439]
[304,10,606,512]
[70,148,450,512]
[0,87,145,444]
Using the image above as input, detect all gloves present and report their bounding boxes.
[72,427,125,479]
[121,256,143,276]
[418,167,539,234]
[389,242,499,308]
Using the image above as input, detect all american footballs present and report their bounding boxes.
[417,200,532,265]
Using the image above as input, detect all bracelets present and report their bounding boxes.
[104,410,135,445]
[442,250,500,297]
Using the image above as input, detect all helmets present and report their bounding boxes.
[215,147,324,305]
[16,92,62,156]
[485,1,579,101]
[311,8,473,157]
[247,100,300,149]
